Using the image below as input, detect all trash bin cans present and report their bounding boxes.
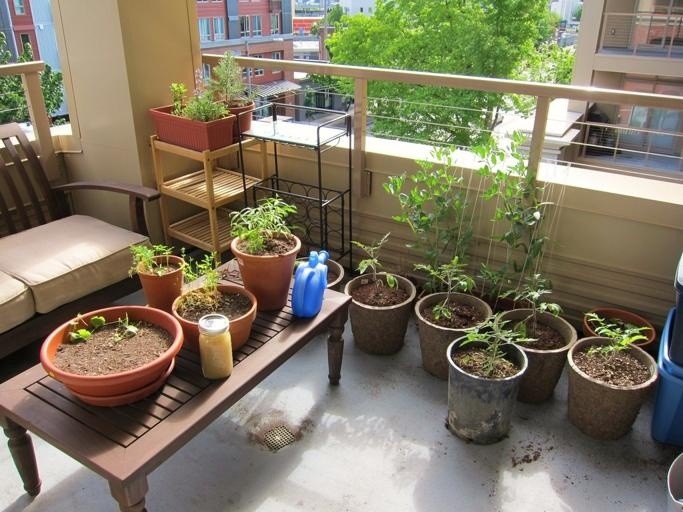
[667,453,683,512]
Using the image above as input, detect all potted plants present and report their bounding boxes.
[161,253,257,351]
[224,190,303,312]
[146,83,235,156]
[293,131,661,445]
[202,46,255,135]
[39,301,184,407]
[124,240,188,310]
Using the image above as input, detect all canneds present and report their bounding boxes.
[198,313,233,379]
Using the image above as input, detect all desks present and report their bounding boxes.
[0,251,351,512]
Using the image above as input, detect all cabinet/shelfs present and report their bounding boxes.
[234,99,357,280]
[147,132,267,274]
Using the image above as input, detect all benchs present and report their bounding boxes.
[0,122,159,364]
